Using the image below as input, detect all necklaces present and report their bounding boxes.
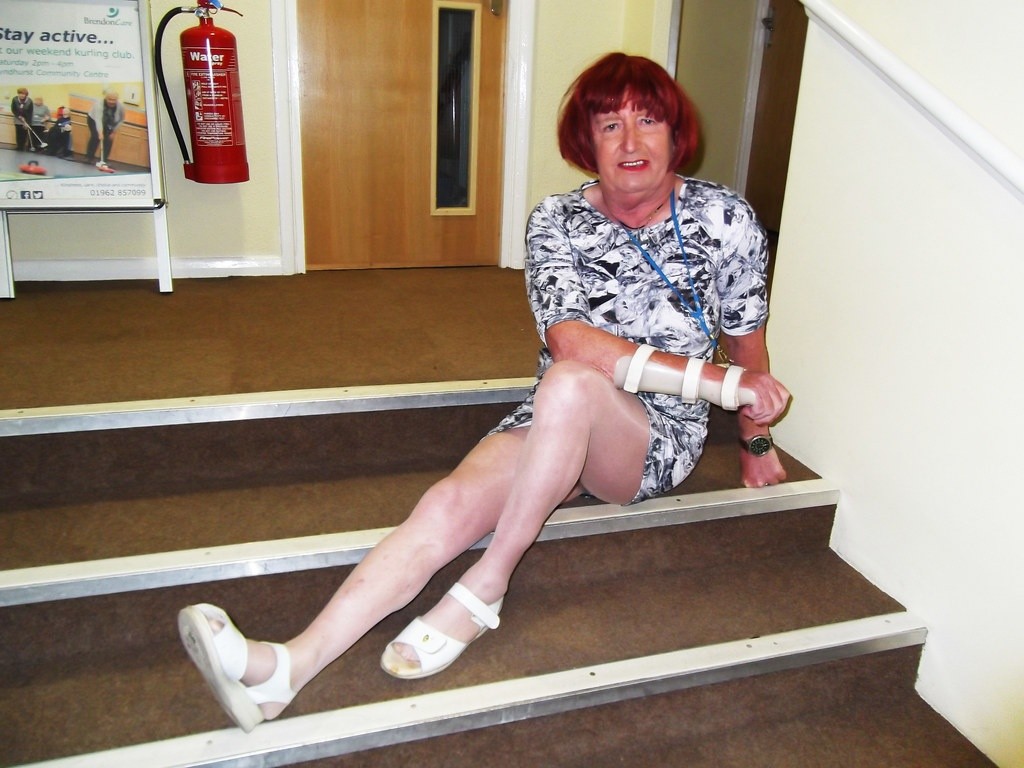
[617,203,664,230]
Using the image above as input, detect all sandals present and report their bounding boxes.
[379,580,502,680]
[179,603,298,733]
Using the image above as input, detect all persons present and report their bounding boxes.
[85,88,126,174]
[11,87,73,156]
[174,49,802,736]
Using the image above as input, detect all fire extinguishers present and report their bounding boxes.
[154,0,249,185]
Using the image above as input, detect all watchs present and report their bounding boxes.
[738,434,774,458]
